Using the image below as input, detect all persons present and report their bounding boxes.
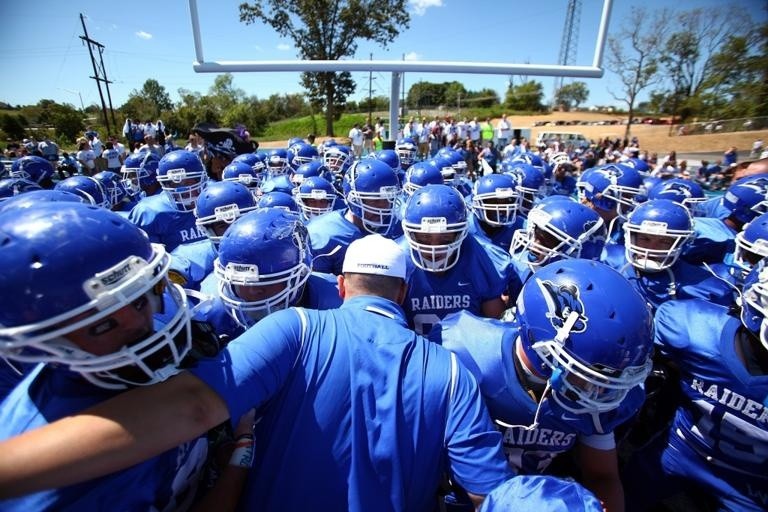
[468,118,481,144]
[390,185,505,337]
[168,181,258,288]
[415,118,430,160]
[305,160,404,310]
[1,233,509,512]
[650,178,705,206]
[0,191,91,204]
[11,156,57,190]
[682,174,768,265]
[431,120,444,156]
[57,153,78,179]
[38,135,60,162]
[244,131,259,153]
[373,116,379,130]
[498,113,510,150]
[455,118,468,138]
[134,119,144,142]
[24,135,39,157]
[307,134,317,147]
[144,119,155,135]
[123,118,135,152]
[396,138,418,168]
[467,174,533,282]
[109,135,126,159]
[519,138,530,154]
[618,200,734,314]
[655,258,767,511]
[501,140,519,158]
[194,128,244,180]
[0,202,256,512]
[186,134,203,153]
[155,120,166,145]
[221,164,258,187]
[113,152,160,210]
[477,474,604,511]
[0,178,45,197]
[586,162,648,244]
[259,192,299,211]
[88,134,105,159]
[128,150,209,252]
[102,141,123,173]
[731,213,768,308]
[536,137,764,171]
[362,118,375,153]
[191,209,342,330]
[402,162,445,196]
[377,119,386,142]
[349,122,364,159]
[482,117,494,143]
[165,134,185,152]
[77,141,99,177]
[405,120,415,140]
[94,172,126,207]
[443,120,456,143]
[425,259,655,511]
[576,168,602,208]
[477,140,502,176]
[465,141,480,169]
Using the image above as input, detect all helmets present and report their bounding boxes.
[445,151,467,183]
[324,146,355,178]
[286,142,320,172]
[733,212,768,272]
[429,157,456,185]
[736,254,768,350]
[288,137,304,148]
[221,162,260,192]
[213,206,313,333]
[648,178,707,217]
[722,172,768,223]
[11,155,55,184]
[258,191,302,217]
[0,189,89,212]
[92,171,127,206]
[0,177,42,202]
[0,201,193,390]
[470,172,519,227]
[622,199,696,273]
[512,151,546,174]
[320,141,336,154]
[621,158,649,177]
[585,162,648,221]
[549,152,570,175]
[121,151,161,196]
[195,181,258,245]
[395,137,418,165]
[576,166,600,204]
[342,158,400,236]
[402,184,469,272]
[402,162,443,203]
[377,149,401,175]
[515,258,655,416]
[636,177,663,201]
[155,149,210,214]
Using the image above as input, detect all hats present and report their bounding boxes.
[341,232,406,280]
[191,121,257,159]
